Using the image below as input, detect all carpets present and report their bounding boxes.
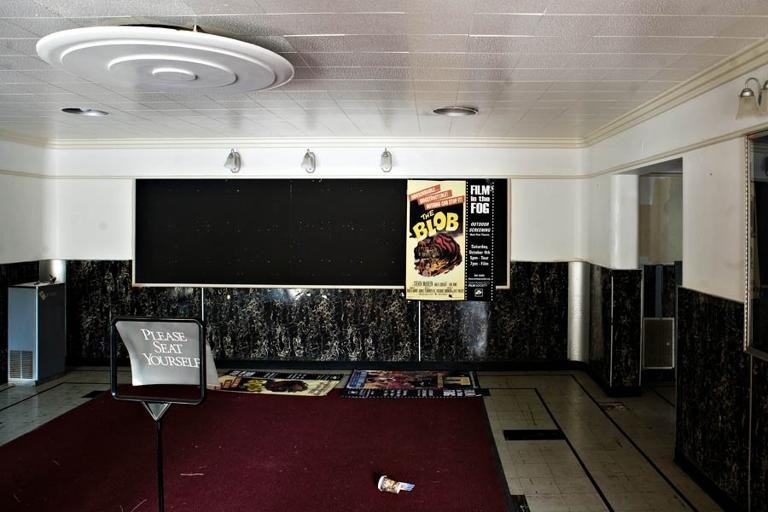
[0,383,533,512]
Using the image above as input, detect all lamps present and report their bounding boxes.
[733,71,768,124]
[33,22,296,97]
[430,103,479,119]
[224,146,393,175]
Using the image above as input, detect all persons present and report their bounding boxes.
[215,376,309,393]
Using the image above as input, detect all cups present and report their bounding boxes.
[378,475,400,494]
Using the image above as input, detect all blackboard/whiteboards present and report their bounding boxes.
[133,176,512,289]
